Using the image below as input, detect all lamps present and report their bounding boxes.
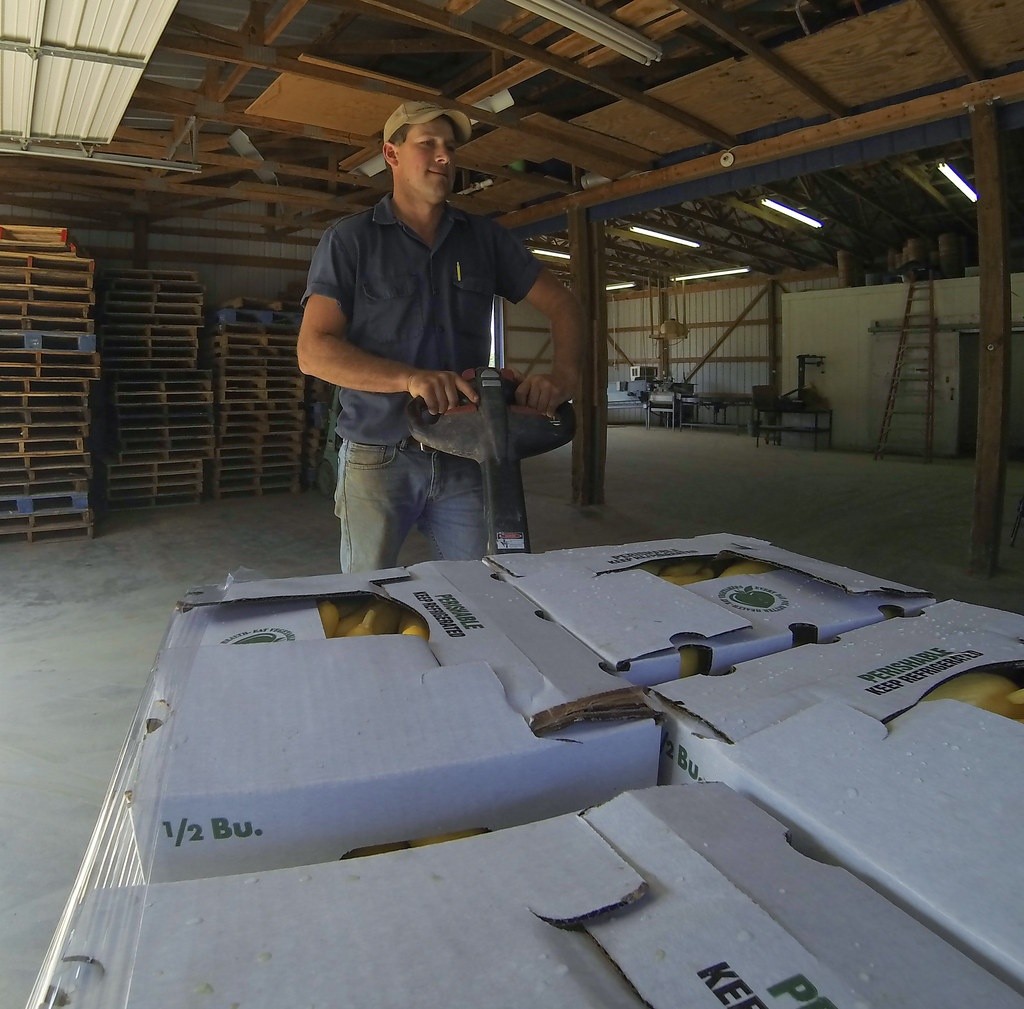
[530,158,978,290]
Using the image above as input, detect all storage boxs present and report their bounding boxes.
[26,531,1023,1009]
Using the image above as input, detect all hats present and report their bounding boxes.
[384,102,471,146]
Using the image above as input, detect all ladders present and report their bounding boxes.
[874,270,941,464]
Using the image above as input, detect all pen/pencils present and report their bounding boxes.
[456,262,462,281]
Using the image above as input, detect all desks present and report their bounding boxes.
[680,393,756,437]
[757,407,833,451]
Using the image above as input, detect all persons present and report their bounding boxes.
[296,101,580,574]
